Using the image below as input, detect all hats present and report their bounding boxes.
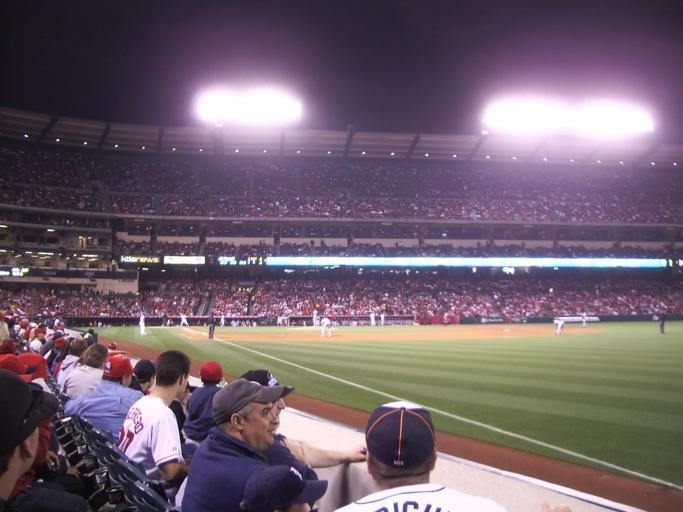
[239,368,295,397]
[0,311,6,321]
[200,360,224,385]
[211,380,284,425]
[102,354,135,378]
[0,338,59,452]
[18,321,65,348]
[134,359,157,383]
[364,401,435,470]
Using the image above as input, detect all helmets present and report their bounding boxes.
[108,342,118,350]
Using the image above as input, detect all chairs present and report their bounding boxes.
[54,415,172,512]
[43,374,69,410]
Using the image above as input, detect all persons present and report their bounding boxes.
[0,145,682,339]
[240,369,367,512]
[332,399,505,512]
[0,339,136,510]
[239,462,329,512]
[133,358,155,393]
[116,349,192,488]
[183,361,225,442]
[181,377,292,512]
[169,380,190,444]
